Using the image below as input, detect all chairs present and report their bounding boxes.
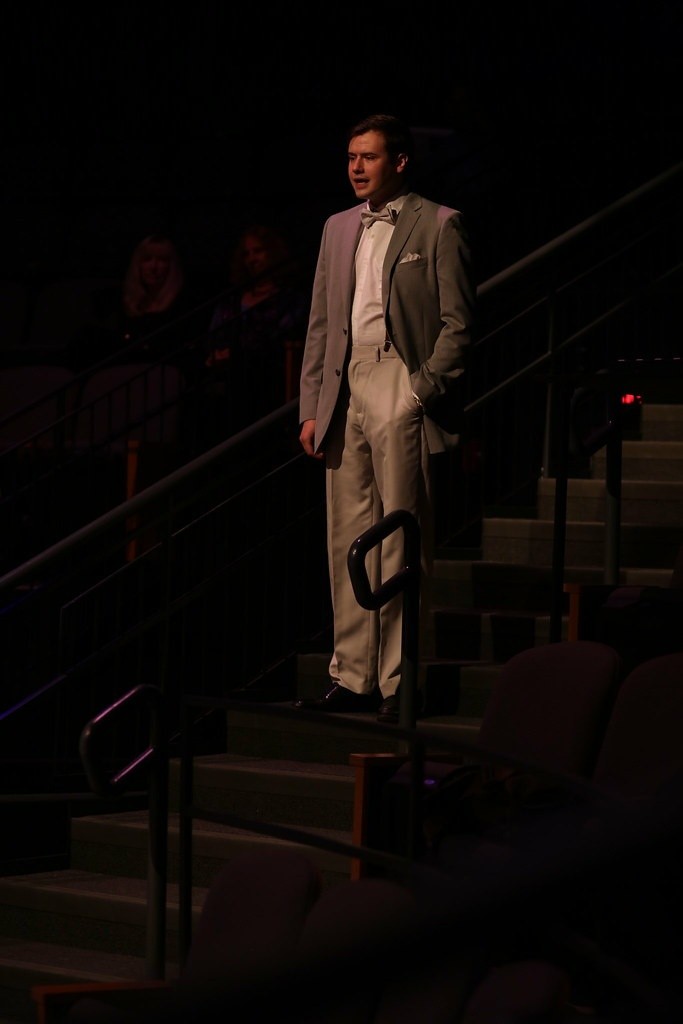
[594,653,683,801]
[181,845,322,983]
[450,641,615,802]
[303,877,467,1024]
[465,961,561,1024]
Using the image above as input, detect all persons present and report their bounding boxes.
[292,112,471,722]
[62,204,293,376]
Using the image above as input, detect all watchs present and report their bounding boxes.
[414,398,421,408]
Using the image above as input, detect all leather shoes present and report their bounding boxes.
[291,684,377,712]
[378,695,400,720]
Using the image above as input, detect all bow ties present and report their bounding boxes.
[359,205,396,229]
